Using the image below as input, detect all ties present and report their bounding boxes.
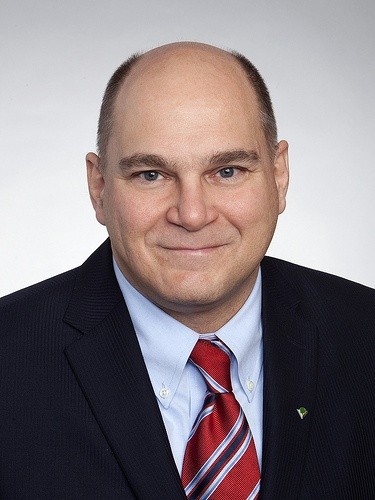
[180,340,261,500]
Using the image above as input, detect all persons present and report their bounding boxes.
[0,41,375,500]
